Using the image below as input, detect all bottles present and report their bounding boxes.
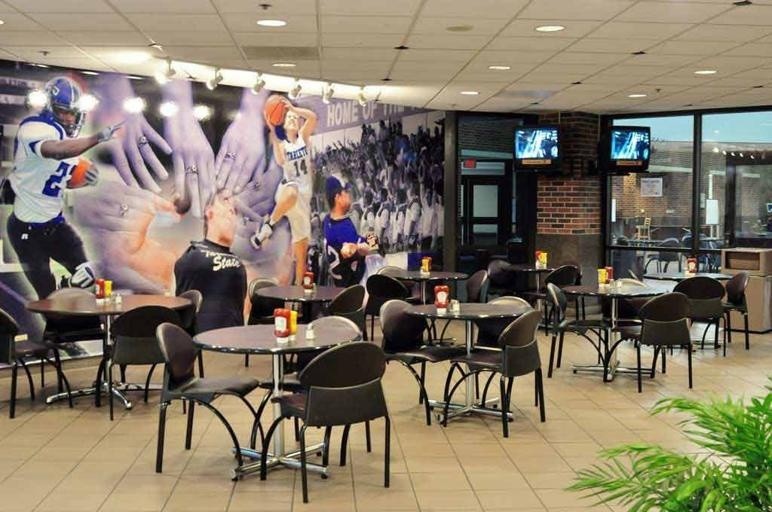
[598,267,613,288]
[304,272,313,294]
[420,257,431,275]
[95,278,122,304]
[535,251,547,270]
[434,285,460,315]
[274,308,315,344]
[688,258,696,276]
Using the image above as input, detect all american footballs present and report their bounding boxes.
[67,159,93,187]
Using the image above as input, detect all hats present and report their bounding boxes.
[324,173,353,196]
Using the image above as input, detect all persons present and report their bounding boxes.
[174,190,247,333]
[539,132,555,154]
[70,74,289,294]
[322,175,378,286]
[310,117,446,253]
[6,76,124,356]
[635,134,650,158]
[250,98,318,282]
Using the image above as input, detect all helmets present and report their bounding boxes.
[38,76,84,141]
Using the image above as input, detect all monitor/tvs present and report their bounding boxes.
[512,124,567,175]
[597,125,650,174]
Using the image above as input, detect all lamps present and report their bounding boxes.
[706,199,720,240]
[155,56,367,106]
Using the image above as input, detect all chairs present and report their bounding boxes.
[466,227,772,389]
[3,231,391,502]
[367,232,548,438]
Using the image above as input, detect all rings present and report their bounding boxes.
[186,165,199,175]
[136,137,148,148]
[253,181,260,191]
[225,153,237,160]
[120,204,128,216]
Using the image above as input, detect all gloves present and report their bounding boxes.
[97,120,126,142]
[84,163,101,188]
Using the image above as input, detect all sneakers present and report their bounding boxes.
[251,213,274,250]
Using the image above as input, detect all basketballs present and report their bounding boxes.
[266,95,287,126]
